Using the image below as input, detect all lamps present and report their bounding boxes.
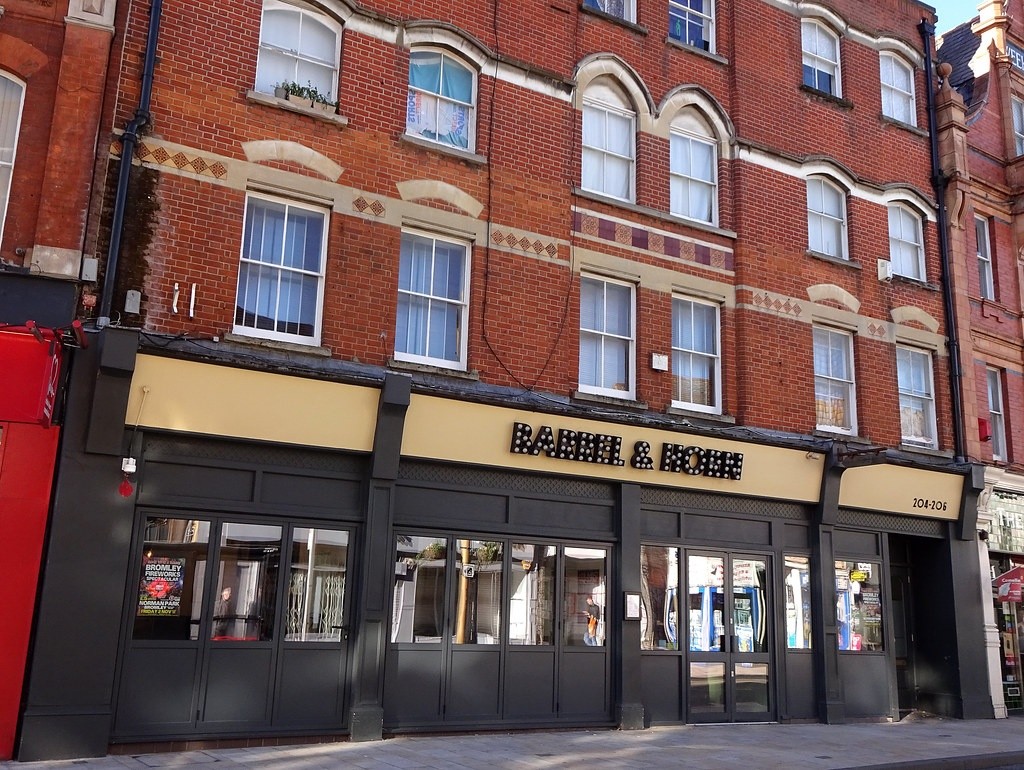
[977,527,989,540]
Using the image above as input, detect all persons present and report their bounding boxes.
[583,596,600,646]
[214,587,236,636]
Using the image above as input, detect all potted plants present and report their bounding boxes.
[416,540,445,559]
[311,87,337,113]
[476,541,500,562]
[270,80,293,99]
[287,81,316,108]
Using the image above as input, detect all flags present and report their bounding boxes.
[997,567,1021,602]
